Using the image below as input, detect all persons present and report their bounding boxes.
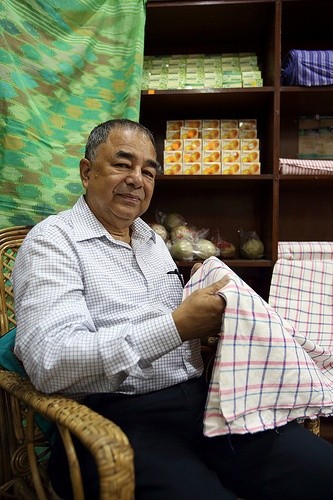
[13,119,333,500]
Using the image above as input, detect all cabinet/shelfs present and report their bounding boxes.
[140,0,333,272]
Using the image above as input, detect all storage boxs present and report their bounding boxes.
[163,119,261,174]
[297,115,333,160]
[142,53,263,90]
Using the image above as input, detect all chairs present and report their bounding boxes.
[0,226,321,500]
